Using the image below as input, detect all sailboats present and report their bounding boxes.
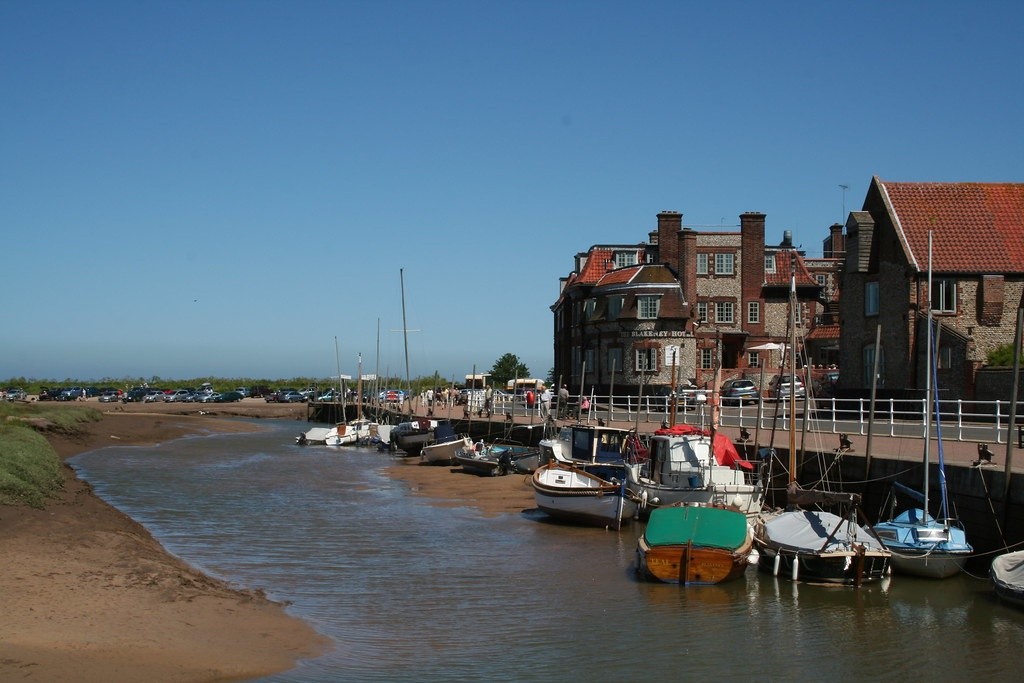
[295,265,1024,610]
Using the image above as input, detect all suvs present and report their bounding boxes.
[250,385,271,398]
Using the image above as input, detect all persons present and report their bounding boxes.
[558,384,569,420]
[426,387,460,406]
[570,395,589,420]
[485,385,492,415]
[540,386,551,423]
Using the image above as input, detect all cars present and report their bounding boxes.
[6,387,28,400]
[657,383,697,412]
[39,383,251,403]
[768,373,805,403]
[720,379,759,406]
[815,372,840,396]
[278,390,307,404]
